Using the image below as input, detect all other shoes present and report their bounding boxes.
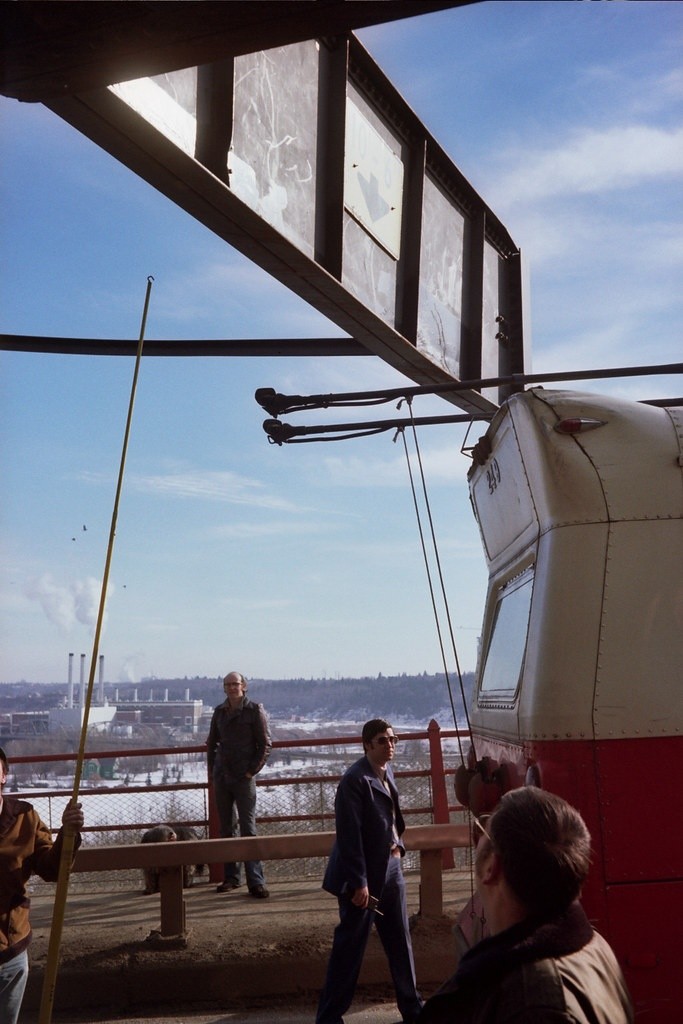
[250,883,269,897]
[217,880,240,892]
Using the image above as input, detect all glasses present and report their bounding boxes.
[473,812,499,853]
[224,682,242,687]
[371,736,399,744]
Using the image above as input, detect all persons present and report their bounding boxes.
[316,718,424,1024]
[407,786,634,1024]
[0,746,85,1024]
[206,673,271,896]
